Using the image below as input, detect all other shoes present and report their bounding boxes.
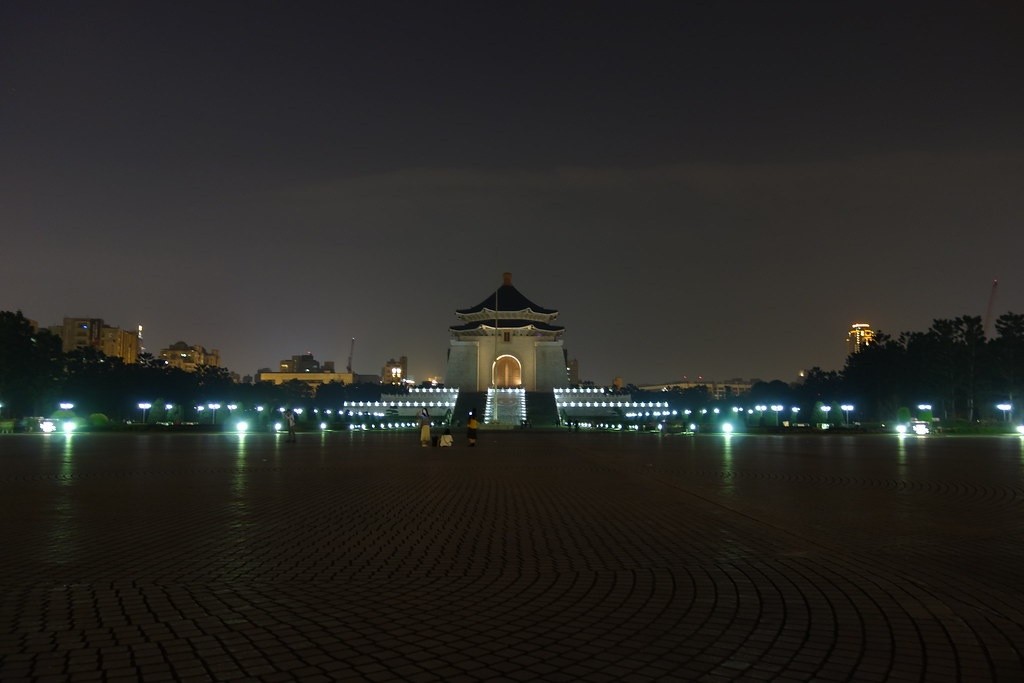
[422,444,429,448]
[292,440,295,443]
[468,443,474,448]
[286,440,291,442]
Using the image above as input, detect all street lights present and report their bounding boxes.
[841,405,854,424]
[997,404,1012,421]
[792,407,800,422]
[821,406,831,421]
[771,405,783,426]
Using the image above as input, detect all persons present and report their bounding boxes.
[466,408,479,448]
[521,416,536,431]
[567,416,573,432]
[440,428,456,449]
[551,415,561,430]
[573,419,580,432]
[285,409,297,442]
[420,408,432,448]
